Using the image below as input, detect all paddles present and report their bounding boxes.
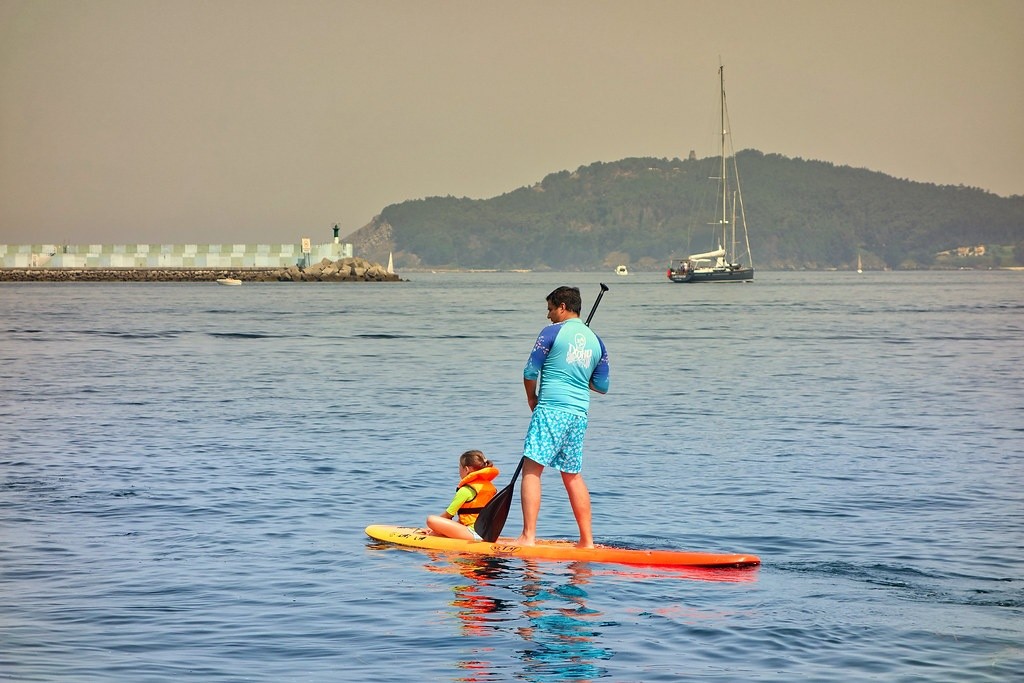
[473,282,610,543]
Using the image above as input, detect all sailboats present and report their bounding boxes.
[665,59,755,283]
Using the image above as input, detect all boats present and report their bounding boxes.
[216,278,243,286]
[614,265,629,277]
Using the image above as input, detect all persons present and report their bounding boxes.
[425,450,500,541]
[680,261,688,275]
[523,286,610,549]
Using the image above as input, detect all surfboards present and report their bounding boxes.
[364,524,759,567]
